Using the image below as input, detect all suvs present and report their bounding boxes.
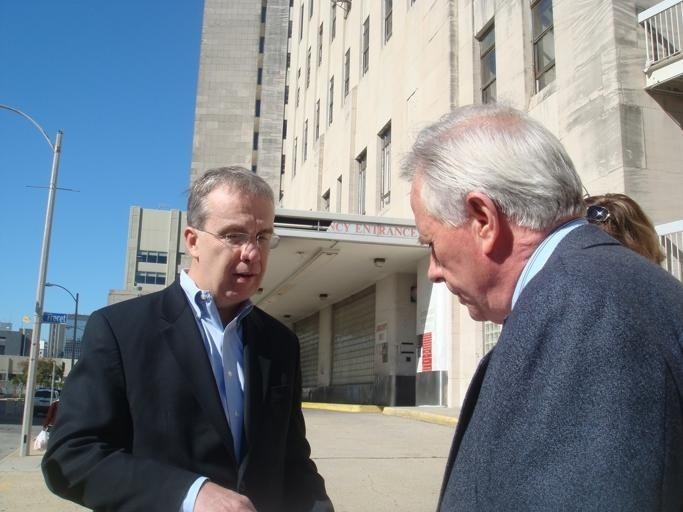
[33,388,59,416]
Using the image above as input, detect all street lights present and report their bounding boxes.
[43,282,78,371]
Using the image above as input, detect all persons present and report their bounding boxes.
[42,399,60,433]
[400,102,683,512]
[584,194,664,265]
[41,170,334,512]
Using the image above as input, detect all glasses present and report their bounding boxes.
[586,206,621,230]
[189,228,280,251]
